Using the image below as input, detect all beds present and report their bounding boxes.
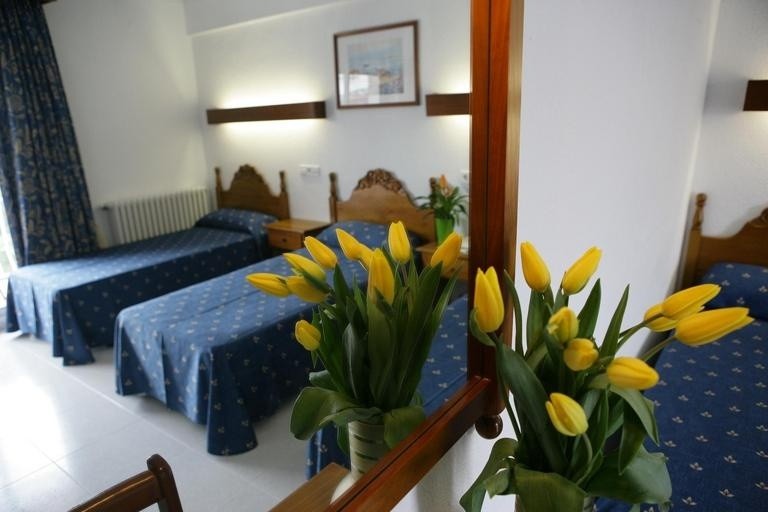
[2,164,289,367]
[112,168,437,457]
[592,192,768,511]
[305,290,469,480]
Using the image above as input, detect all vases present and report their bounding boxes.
[436,217,454,246]
[350,418,408,475]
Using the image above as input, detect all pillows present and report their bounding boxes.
[314,220,422,250]
[697,259,768,320]
[193,207,278,238]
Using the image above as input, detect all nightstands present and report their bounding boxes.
[261,218,331,251]
[415,238,469,304]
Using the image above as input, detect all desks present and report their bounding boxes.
[265,459,351,511]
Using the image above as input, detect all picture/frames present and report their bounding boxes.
[333,19,422,111]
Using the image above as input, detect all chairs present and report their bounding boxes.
[69,452,184,511]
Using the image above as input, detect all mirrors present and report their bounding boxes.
[0,1,524,509]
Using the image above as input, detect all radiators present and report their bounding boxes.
[104,186,209,246]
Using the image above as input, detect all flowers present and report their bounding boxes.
[245,218,464,409]
[457,239,760,512]
[414,173,467,217]
